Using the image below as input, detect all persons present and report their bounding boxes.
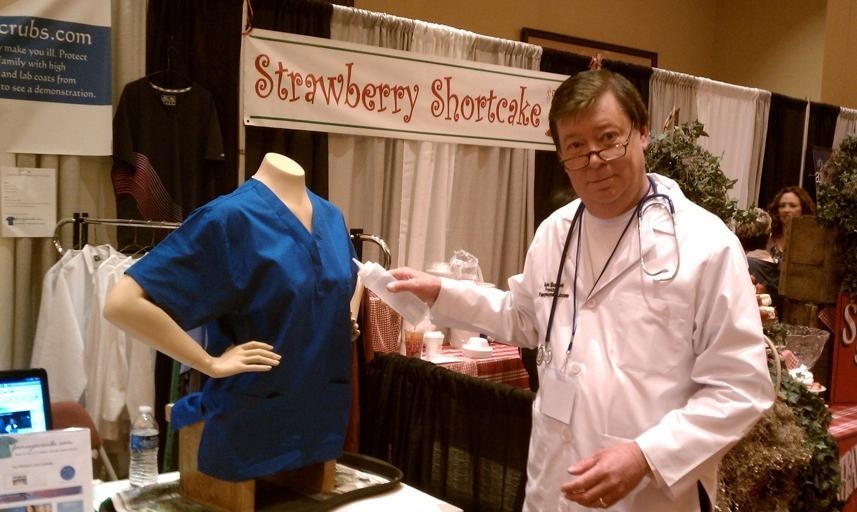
[385,69,777,512]
[736,208,780,306]
[104,153,365,483]
[767,187,815,264]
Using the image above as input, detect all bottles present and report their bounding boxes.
[128,406,160,511]
[350,253,427,324]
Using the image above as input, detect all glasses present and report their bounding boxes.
[559,121,635,170]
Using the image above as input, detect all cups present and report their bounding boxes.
[423,332,445,358]
[402,329,423,359]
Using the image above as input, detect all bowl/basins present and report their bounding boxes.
[461,336,492,358]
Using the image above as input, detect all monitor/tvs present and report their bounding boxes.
[0,368,53,435]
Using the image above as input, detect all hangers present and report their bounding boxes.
[148,46,188,81]
[72,220,157,259]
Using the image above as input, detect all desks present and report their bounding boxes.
[420,341,530,389]
[95,462,464,511]
[824,398,856,511]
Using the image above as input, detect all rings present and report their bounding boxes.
[600,497,608,508]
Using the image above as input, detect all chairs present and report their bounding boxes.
[51,400,118,481]
